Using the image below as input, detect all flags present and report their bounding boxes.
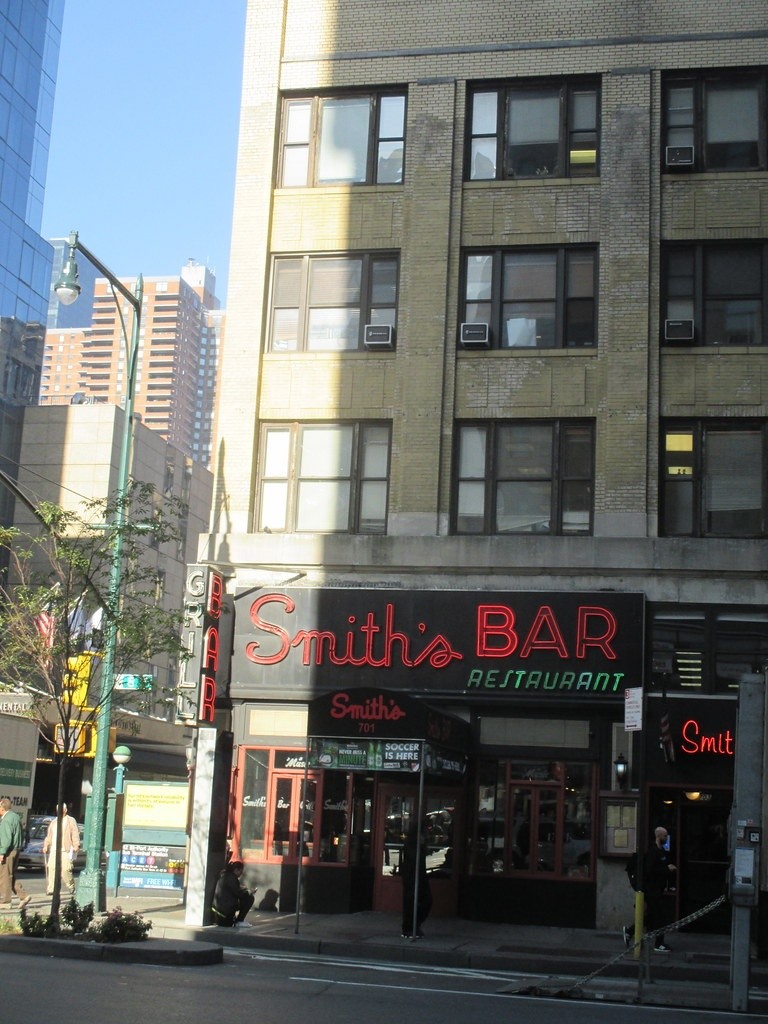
[32,589,58,668]
[62,603,86,655]
[84,607,106,665]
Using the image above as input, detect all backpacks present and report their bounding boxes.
[624,849,655,891]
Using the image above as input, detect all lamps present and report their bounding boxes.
[614,753,629,790]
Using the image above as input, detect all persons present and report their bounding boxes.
[623,827,678,953]
[211,861,254,927]
[44,802,81,894]
[402,822,432,939]
[0,797,32,909]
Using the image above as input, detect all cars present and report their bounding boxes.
[16,817,108,872]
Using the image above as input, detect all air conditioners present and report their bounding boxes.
[363,325,396,349]
[461,322,491,349]
[666,146,695,170]
[664,319,695,344]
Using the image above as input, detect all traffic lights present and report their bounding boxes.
[62,655,90,707]
[54,720,86,754]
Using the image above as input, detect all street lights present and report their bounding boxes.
[104,746,132,887]
[53,229,143,922]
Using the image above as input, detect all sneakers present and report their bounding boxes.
[654,944,671,952]
[623,926,632,947]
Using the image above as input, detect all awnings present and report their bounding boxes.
[294,687,474,940]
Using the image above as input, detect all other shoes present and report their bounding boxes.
[0,902,12,909]
[18,896,31,909]
[401,930,425,939]
[233,921,252,928]
[68,882,75,894]
[46,888,54,895]
